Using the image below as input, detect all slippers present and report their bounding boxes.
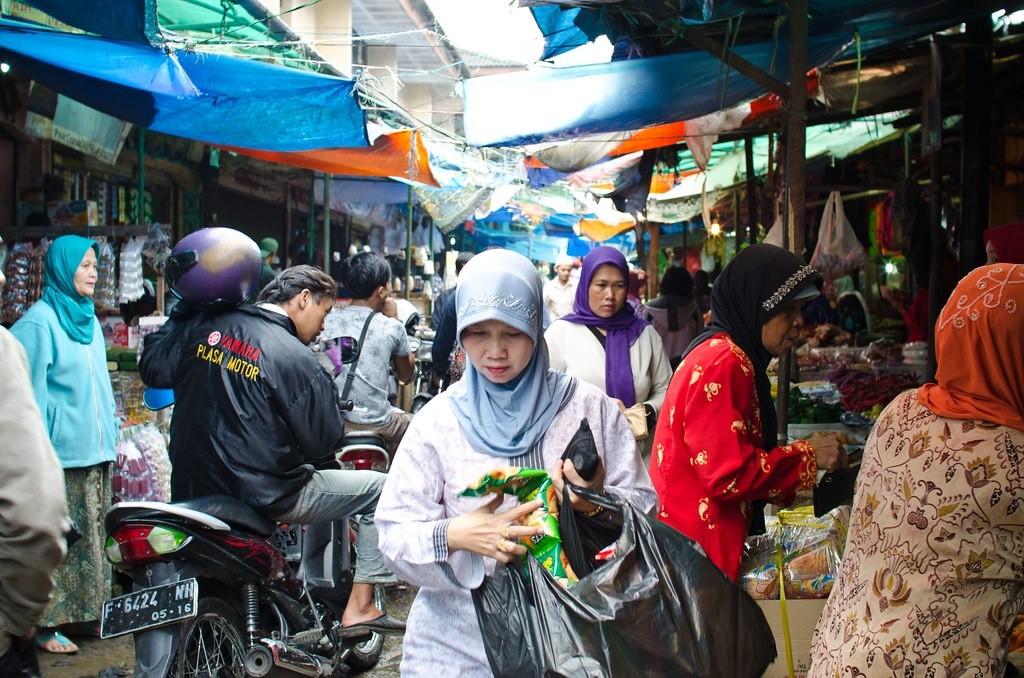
[338,611,406,639]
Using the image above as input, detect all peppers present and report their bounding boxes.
[838,370,922,411]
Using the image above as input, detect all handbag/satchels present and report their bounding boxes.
[471,480,777,678]
[810,192,869,272]
[762,215,783,248]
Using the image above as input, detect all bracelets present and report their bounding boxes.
[581,489,611,520]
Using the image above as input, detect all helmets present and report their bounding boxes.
[164,227,264,310]
[396,299,420,336]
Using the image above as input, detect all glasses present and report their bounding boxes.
[0,241,8,268]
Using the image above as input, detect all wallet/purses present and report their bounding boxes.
[812,448,865,518]
[624,403,651,440]
[560,417,599,484]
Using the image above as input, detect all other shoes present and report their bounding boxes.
[35,619,101,652]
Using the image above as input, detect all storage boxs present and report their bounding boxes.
[755,599,827,678]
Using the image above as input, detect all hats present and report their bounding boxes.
[554,254,573,267]
[455,252,475,269]
[260,238,278,257]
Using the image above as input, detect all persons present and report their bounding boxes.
[138,220,1024,678]
[8,235,121,653]
[0,238,72,678]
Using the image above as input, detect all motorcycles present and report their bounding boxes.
[410,327,459,417]
[101,388,389,678]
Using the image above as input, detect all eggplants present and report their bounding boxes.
[821,362,866,385]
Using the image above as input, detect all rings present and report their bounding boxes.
[502,525,512,539]
[497,540,508,553]
